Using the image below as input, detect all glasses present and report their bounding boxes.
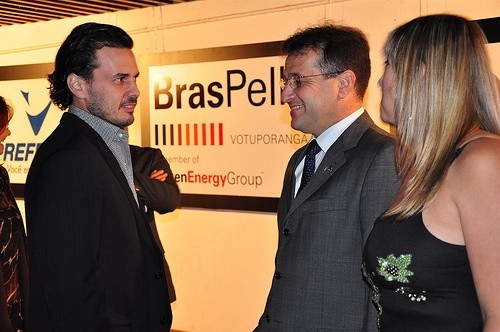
[280,70,346,91]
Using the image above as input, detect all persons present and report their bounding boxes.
[252,14,500,332]
[124,126,180,302]
[0,96,32,332]
[25,23,182,332]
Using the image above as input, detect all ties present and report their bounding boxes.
[294,138,322,196]
